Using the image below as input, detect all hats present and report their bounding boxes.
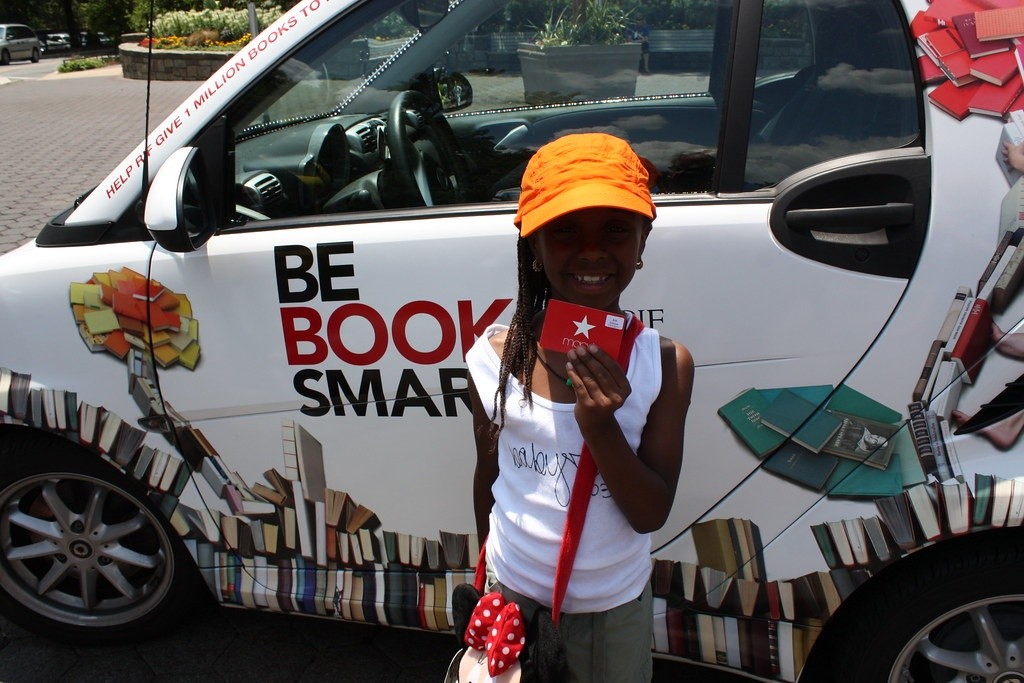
[514,131,658,237]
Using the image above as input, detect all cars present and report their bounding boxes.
[0,1,1024,683]
[0,24,42,65]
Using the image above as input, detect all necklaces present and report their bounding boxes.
[536,310,626,388]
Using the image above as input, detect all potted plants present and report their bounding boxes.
[516,0,645,109]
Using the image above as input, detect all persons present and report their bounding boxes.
[465,134,694,683]
[627,12,651,72]
[1002,139,1024,173]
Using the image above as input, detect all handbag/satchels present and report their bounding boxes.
[440,582,564,683]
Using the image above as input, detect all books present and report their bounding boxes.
[1,0,1024,683]
